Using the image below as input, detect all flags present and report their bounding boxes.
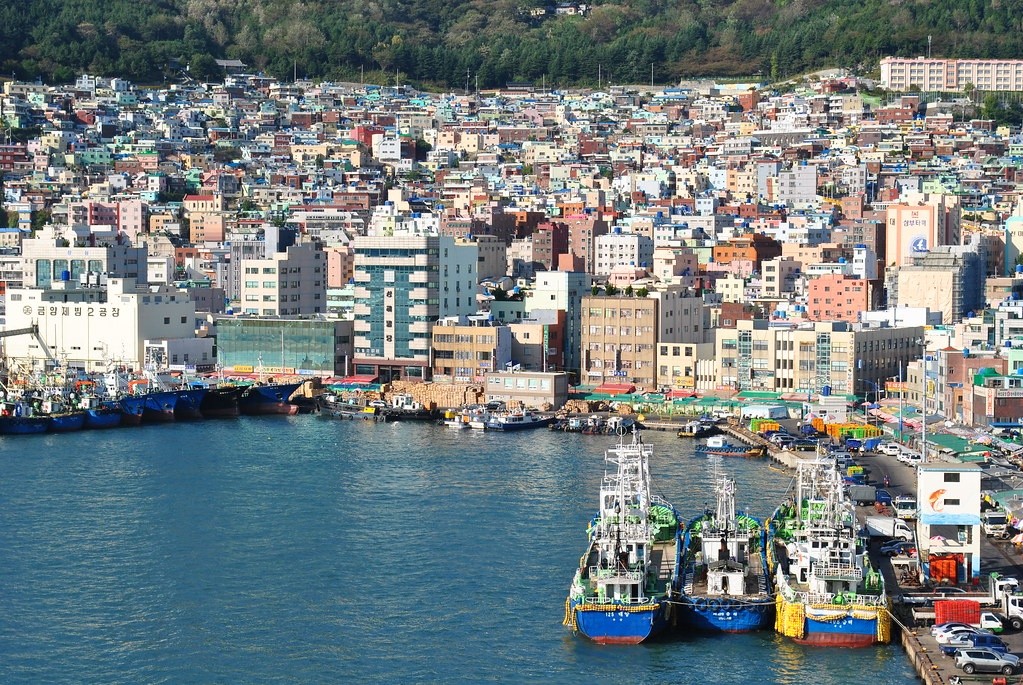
[505,361,512,366]
[513,364,520,370]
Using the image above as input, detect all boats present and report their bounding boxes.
[565,423,683,647]
[676,420,702,437]
[443,398,624,436]
[763,435,892,649]
[676,457,774,634]
[0,323,432,436]
[700,414,719,425]
[694,436,766,457]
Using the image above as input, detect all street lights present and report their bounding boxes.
[858,378,878,427]
[916,337,932,463]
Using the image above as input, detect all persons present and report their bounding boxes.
[780,504,786,516]
[797,421,801,431]
[704,503,708,515]
[884,474,890,481]
[829,437,834,443]
[786,498,792,508]
[850,450,854,457]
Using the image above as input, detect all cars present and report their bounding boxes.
[765,424,1023,676]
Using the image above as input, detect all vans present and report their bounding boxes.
[713,409,735,420]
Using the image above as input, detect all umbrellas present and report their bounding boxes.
[804,413,817,419]
[1011,534,1023,543]
[931,535,946,541]
[1013,519,1023,529]
